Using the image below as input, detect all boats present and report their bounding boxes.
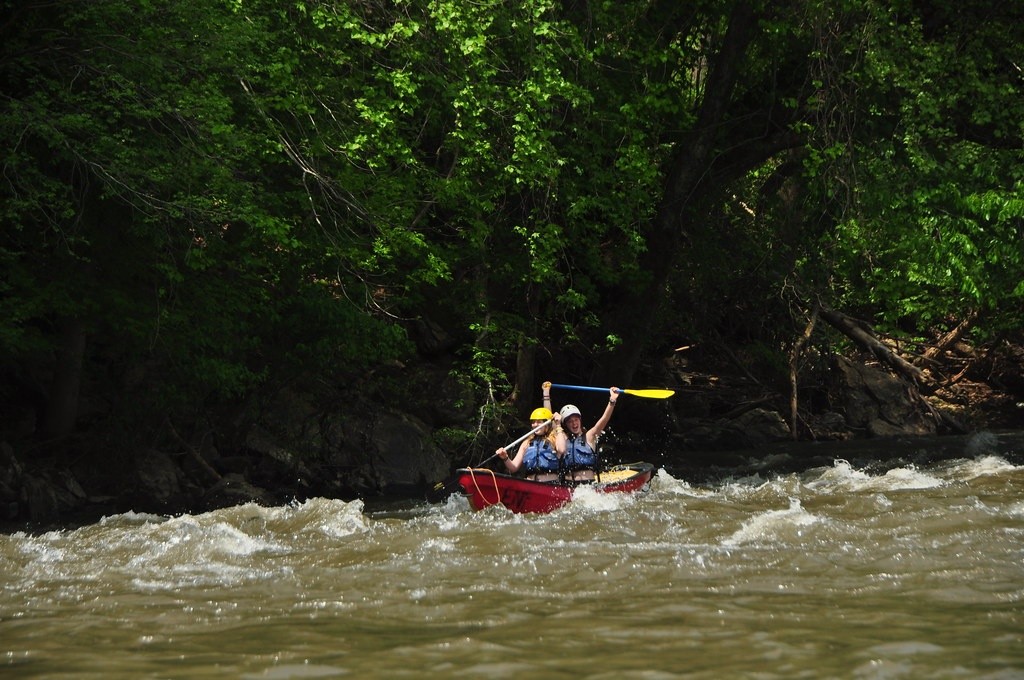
[456,461,654,514]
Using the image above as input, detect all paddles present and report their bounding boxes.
[422,417,553,503]
[545,382,675,399]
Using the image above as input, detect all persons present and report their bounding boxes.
[495,407,566,486]
[542,381,620,488]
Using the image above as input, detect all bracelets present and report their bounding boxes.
[609,399,616,405]
[556,425,564,433]
[503,457,508,461]
[542,395,550,400]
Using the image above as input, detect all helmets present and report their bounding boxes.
[530,408,553,420]
[560,404,581,423]
